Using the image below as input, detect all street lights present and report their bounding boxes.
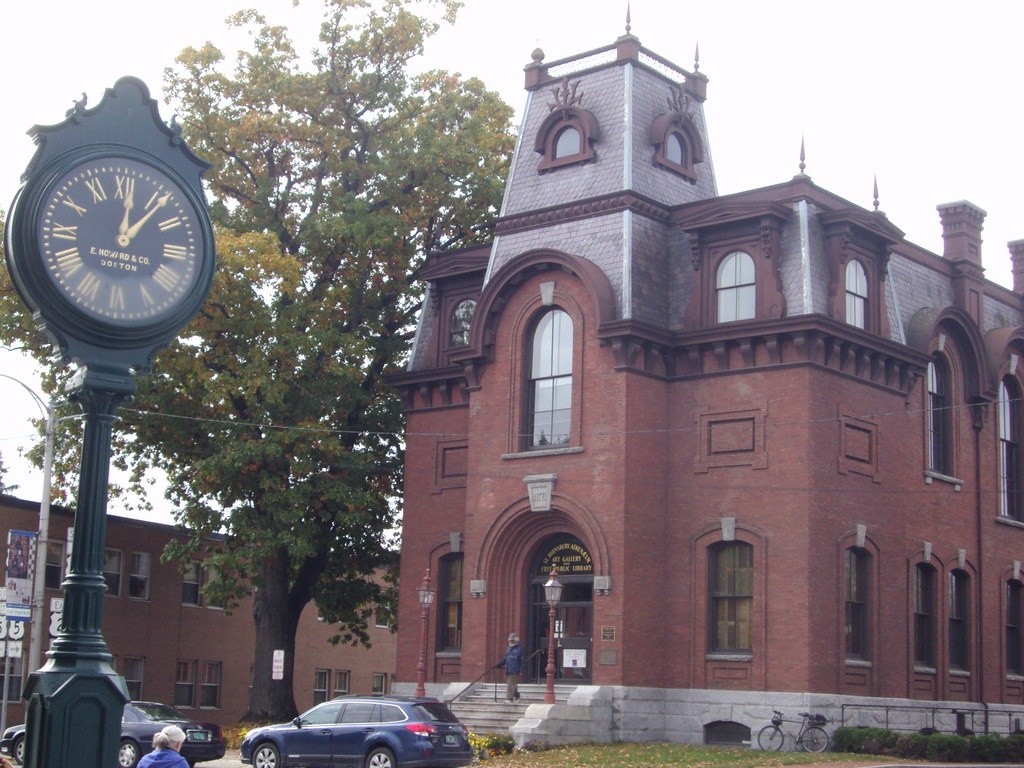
[413,568,436,697]
[542,563,564,705]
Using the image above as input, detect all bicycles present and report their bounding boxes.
[758,710,829,754]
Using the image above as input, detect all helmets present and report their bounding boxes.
[772,716,782,726]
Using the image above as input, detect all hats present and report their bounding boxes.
[509,633,518,638]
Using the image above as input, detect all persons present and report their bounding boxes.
[493,633,525,704]
[137,725,192,768]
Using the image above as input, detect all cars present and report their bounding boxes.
[0,700,225,768]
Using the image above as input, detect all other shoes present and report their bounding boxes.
[504,700,513,704]
[513,697,519,704]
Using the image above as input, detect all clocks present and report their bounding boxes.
[33,149,210,331]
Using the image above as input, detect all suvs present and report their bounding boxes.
[240,692,474,768]
